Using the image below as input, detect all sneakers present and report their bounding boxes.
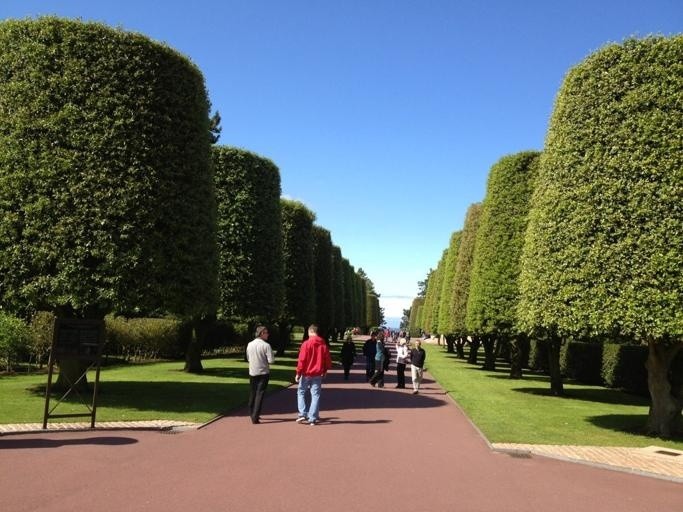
[369,379,375,387]
[297,416,316,426]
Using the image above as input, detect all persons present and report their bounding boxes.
[362,327,425,394]
[246,326,275,424]
[294,323,331,425]
[339,335,358,380]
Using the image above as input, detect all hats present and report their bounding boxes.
[255,326,267,337]
